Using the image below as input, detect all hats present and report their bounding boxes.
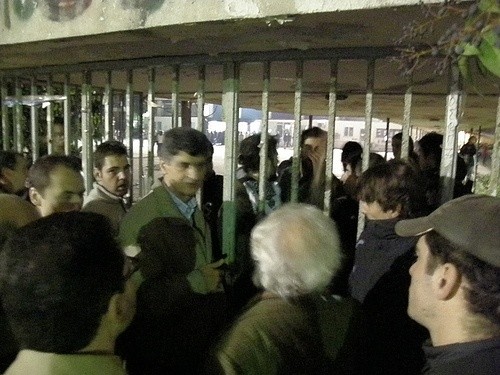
[394,194,500,268]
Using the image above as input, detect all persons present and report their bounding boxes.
[348,157,428,375]
[0,117,130,245]
[405,199,500,375]
[203,127,491,275]
[201,203,368,375]
[1,210,137,375]
[119,127,224,375]
